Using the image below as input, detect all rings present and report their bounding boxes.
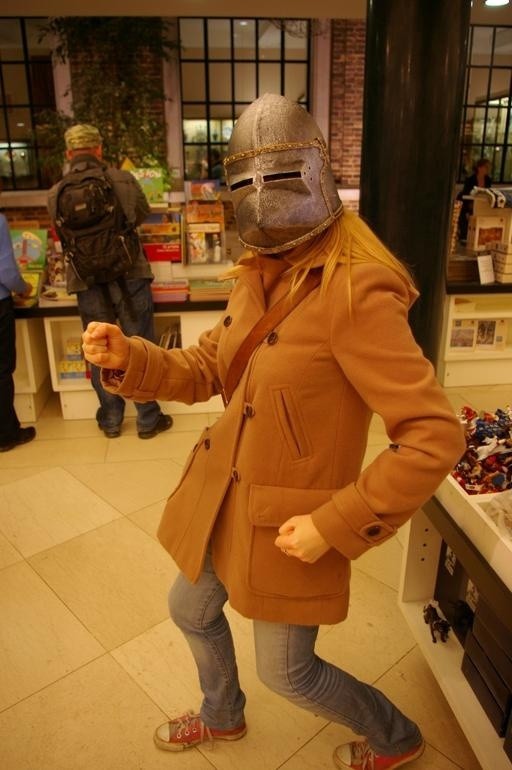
[283,547,290,556]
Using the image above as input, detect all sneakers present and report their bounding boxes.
[332,737,426,770]
[153,711,247,752]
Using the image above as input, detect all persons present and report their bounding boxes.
[76,91,472,768]
[43,121,179,441]
[457,156,495,246]
[1,204,37,455]
[203,148,226,181]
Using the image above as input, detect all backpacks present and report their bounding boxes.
[53,165,140,287]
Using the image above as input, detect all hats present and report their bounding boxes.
[63,124,104,151]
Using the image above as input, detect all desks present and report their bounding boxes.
[398,476,511,770]
[433,270,512,388]
[10,295,231,423]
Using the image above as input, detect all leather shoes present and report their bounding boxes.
[97,406,123,437]
[0,425,37,453]
[138,414,173,439]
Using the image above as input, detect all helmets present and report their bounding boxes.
[223,92,345,254]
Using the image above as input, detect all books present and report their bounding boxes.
[39,292,80,309]
[157,325,179,349]
[10,226,49,273]
[13,297,38,310]
[18,273,43,300]
[140,199,238,305]
